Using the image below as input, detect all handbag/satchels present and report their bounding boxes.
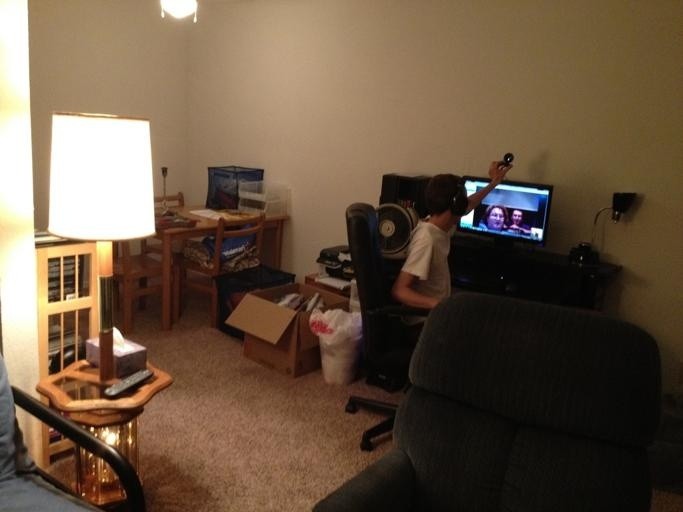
[200,223,257,264]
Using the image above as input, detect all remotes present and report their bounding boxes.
[104,367,154,397]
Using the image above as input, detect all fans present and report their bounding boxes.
[375,201,417,258]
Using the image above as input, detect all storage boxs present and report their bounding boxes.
[205,165,265,210]
[224,281,350,377]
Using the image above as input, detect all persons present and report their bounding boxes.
[390,160,513,332]
[478,204,509,233]
[507,209,532,237]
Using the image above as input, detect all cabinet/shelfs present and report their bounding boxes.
[1,231,117,471]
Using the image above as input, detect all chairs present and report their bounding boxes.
[178,212,266,328]
[346,202,439,452]
[138,191,185,311]
[312,291,662,511]
[0,341,145,511]
[112,238,179,333]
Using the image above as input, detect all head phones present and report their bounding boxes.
[450,176,468,216]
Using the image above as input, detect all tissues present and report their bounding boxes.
[85,325,149,379]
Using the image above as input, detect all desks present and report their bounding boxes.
[306,271,350,297]
[448,233,620,312]
[140,204,290,330]
[36,358,174,507]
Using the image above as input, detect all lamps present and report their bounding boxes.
[571,193,637,262]
[155,0,198,23]
[47,111,156,382]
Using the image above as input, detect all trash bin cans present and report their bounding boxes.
[319,323,362,385]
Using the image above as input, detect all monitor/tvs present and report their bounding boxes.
[457,176,553,247]
